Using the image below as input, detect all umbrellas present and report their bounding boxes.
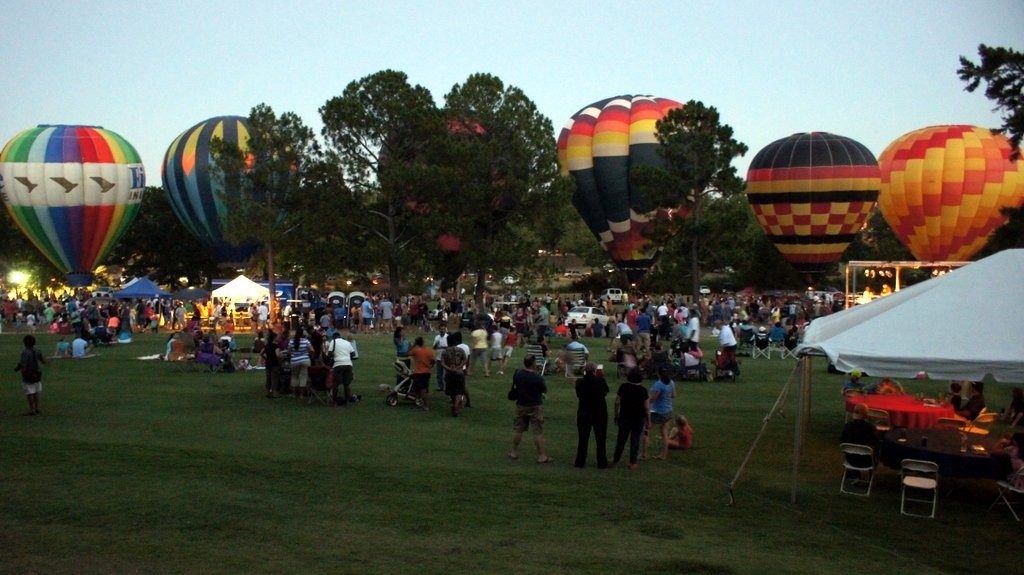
[175,287,210,300]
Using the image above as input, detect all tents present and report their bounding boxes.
[790,247,1024,505]
[212,275,270,317]
[112,277,174,318]
[120,277,160,289]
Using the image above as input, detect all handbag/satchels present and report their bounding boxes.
[323,339,335,368]
[508,369,518,401]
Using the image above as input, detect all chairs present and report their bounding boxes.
[853,402,892,433]
[739,328,785,360]
[650,350,675,376]
[171,345,225,375]
[937,405,998,436]
[715,352,739,382]
[617,351,640,375]
[307,368,333,404]
[565,347,586,379]
[526,344,554,376]
[840,443,879,498]
[987,466,1024,522]
[901,459,939,518]
[684,352,705,382]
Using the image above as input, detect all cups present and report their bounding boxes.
[899,428,907,439]
[921,437,928,448]
[960,436,968,452]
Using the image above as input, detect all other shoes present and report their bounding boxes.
[637,455,647,460]
[500,372,503,374]
[451,406,458,417]
[608,460,616,465]
[483,369,490,373]
[465,372,471,376]
[485,375,489,377]
[652,454,664,460]
[496,371,500,374]
[630,464,638,470]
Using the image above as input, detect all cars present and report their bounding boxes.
[566,306,610,329]
[501,276,514,287]
[91,287,116,299]
[564,270,584,279]
[699,285,711,296]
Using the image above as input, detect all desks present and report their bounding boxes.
[847,394,955,431]
[883,428,1008,499]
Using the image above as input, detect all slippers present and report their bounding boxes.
[507,452,518,459]
[538,457,554,464]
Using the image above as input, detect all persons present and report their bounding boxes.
[656,416,693,450]
[949,383,962,411]
[862,283,892,304]
[0,285,843,418]
[840,369,873,396]
[637,365,677,461]
[840,403,881,487]
[955,381,985,421]
[608,350,651,470]
[1000,387,1024,428]
[992,432,1024,489]
[507,353,554,464]
[14,335,52,416]
[574,363,610,469]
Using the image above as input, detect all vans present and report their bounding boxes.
[600,288,623,305]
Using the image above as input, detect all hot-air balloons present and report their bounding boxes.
[875,125,1024,262]
[745,131,881,291]
[0,123,146,301]
[378,117,509,302]
[161,115,297,283]
[557,94,695,289]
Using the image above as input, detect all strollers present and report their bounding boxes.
[385,357,426,407]
